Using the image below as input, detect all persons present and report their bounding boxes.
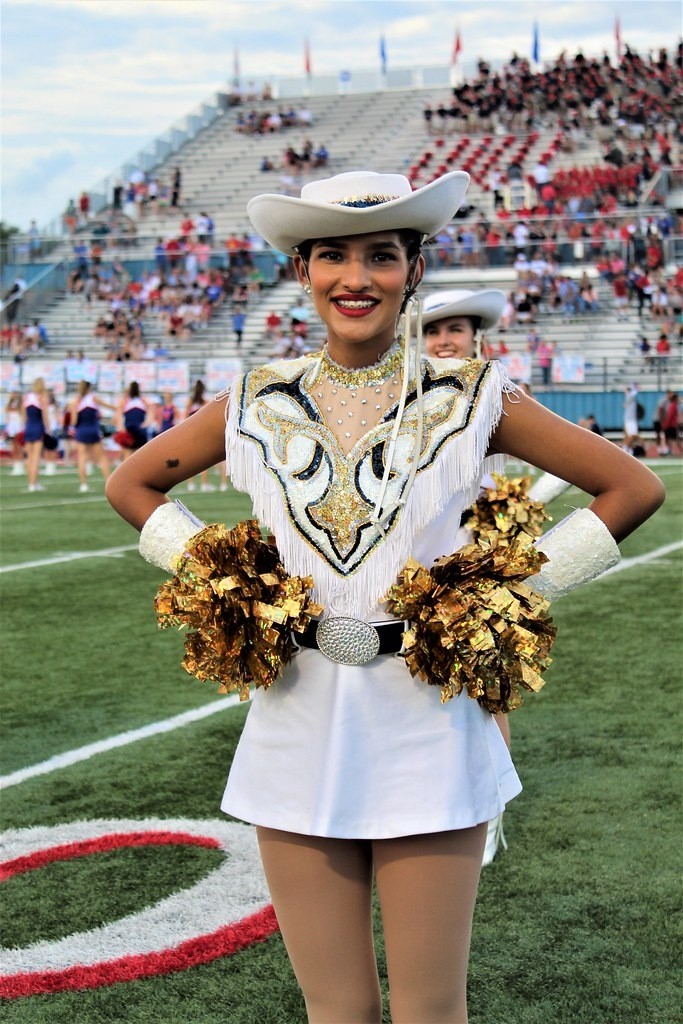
[405,289,573,868]
[184,380,228,492]
[21,378,53,493]
[0,40,683,454]
[68,381,118,492]
[113,381,150,456]
[106,171,666,1024]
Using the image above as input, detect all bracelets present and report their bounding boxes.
[69,425,75,429]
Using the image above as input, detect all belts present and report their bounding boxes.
[294,615,411,666]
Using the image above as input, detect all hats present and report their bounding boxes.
[399,288,506,336]
[246,170,470,258]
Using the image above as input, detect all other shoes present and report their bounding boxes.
[186,482,195,493]
[78,483,89,493]
[219,482,229,493]
[199,483,217,493]
[29,481,47,491]
[481,812,507,867]
